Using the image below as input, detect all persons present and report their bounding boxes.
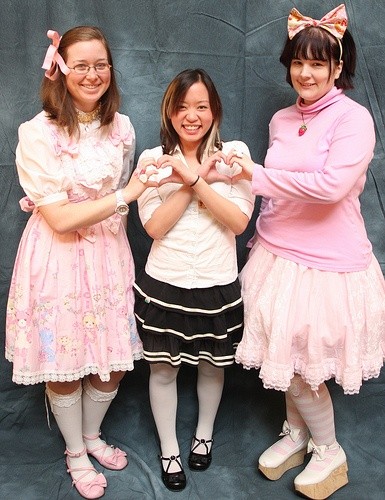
[5,26,159,498]
[226,3,385,500]
[134,68,255,491]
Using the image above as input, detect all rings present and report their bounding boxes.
[134,164,147,183]
[233,148,243,157]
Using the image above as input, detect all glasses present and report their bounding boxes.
[67,63,112,74]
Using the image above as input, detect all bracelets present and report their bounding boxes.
[190,175,200,187]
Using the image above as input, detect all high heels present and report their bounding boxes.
[258,420,307,480]
[294,438,348,500]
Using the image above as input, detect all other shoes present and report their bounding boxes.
[64,444,107,500]
[82,432,128,470]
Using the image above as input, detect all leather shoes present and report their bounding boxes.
[160,454,186,491]
[188,433,214,470]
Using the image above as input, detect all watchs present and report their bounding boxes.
[115,190,129,216]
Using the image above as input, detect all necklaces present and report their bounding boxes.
[299,108,321,137]
